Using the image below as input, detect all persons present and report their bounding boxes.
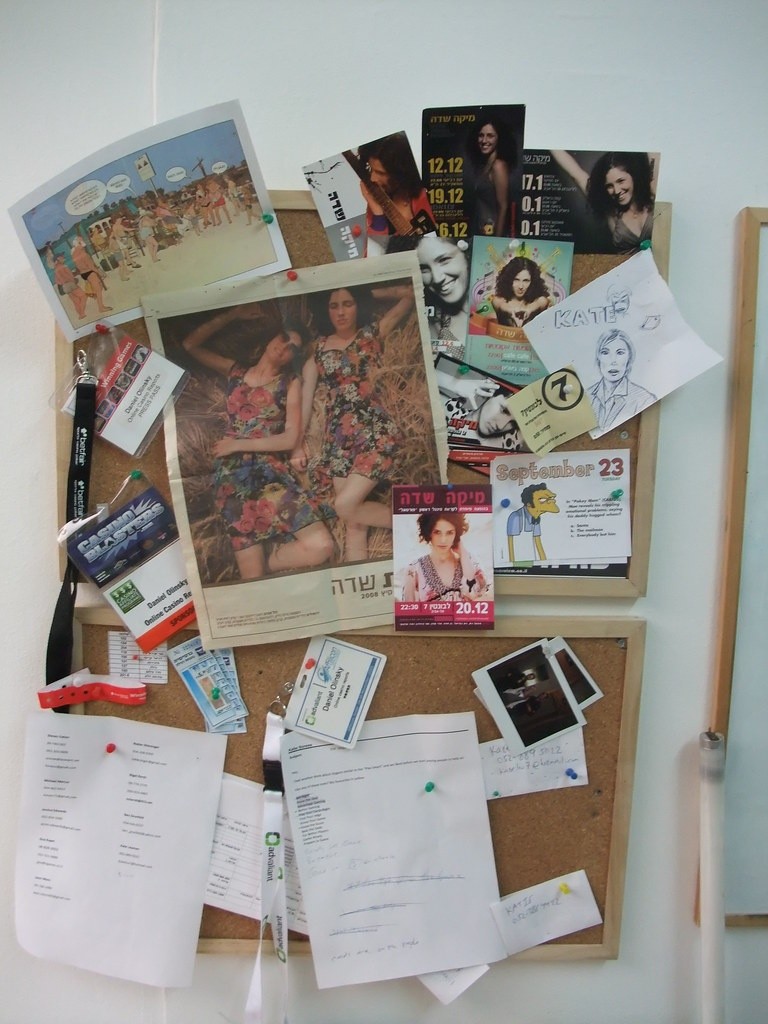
[404,510,488,603]
[550,150,655,253]
[485,255,551,326]
[385,235,472,362]
[357,130,436,250]
[433,368,525,451]
[494,669,549,720]
[467,119,511,234]
[184,285,415,583]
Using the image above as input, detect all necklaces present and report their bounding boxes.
[629,206,639,219]
